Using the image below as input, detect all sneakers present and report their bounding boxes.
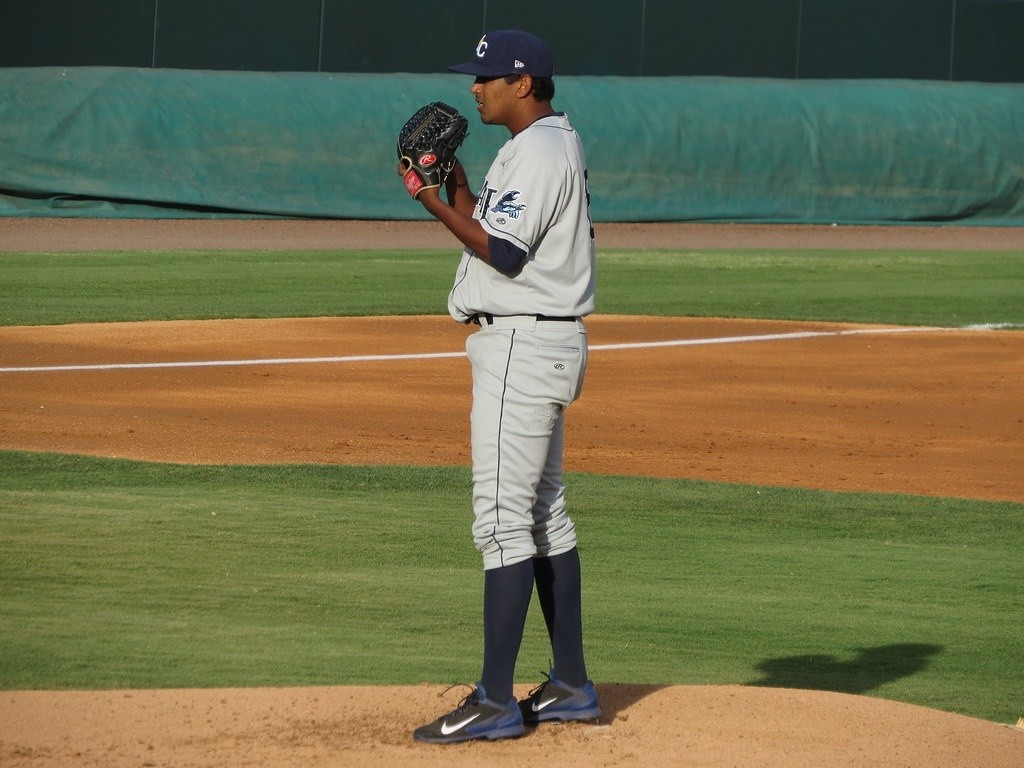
[413,684,526,744]
[518,669,601,724]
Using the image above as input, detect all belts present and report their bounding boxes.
[472,313,575,324]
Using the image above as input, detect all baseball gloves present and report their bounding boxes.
[396,101,471,201]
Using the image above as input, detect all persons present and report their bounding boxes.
[397,27,600,744]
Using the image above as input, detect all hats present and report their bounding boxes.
[447,30,554,79]
